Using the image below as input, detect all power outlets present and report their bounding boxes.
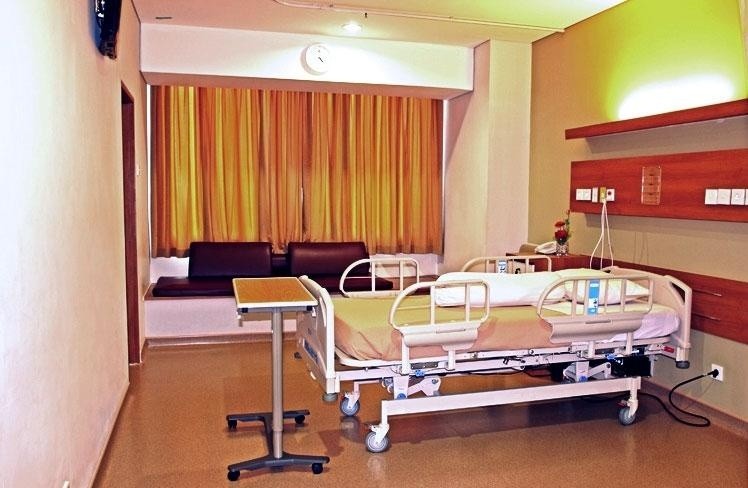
[712,364,724,381]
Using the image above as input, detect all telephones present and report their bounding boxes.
[534,241,556,254]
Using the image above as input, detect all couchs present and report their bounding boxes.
[144,239,393,349]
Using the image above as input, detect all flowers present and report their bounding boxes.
[554,209,573,243]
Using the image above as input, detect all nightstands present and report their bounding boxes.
[507,252,590,272]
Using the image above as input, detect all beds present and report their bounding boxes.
[297,254,693,451]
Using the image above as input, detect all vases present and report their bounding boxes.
[555,242,569,257]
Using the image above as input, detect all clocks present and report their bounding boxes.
[303,43,331,75]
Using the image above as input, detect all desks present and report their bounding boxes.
[226,277,330,482]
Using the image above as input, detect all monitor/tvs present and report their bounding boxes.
[94,0,123,59]
